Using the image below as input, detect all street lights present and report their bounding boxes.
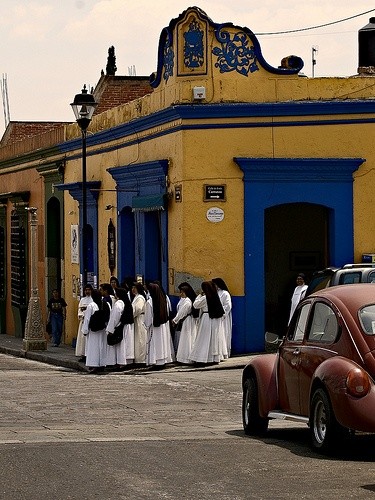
[69,84,99,296]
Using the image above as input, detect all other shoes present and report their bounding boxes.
[132,363,145,369]
[78,356,85,362]
[108,365,130,371]
[195,363,215,367]
[89,367,104,374]
[149,365,163,370]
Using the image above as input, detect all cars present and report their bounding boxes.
[241,283,375,451]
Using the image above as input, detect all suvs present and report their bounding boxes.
[317,263,375,291]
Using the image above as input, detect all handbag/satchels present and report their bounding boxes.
[89,310,108,331]
[106,325,123,345]
